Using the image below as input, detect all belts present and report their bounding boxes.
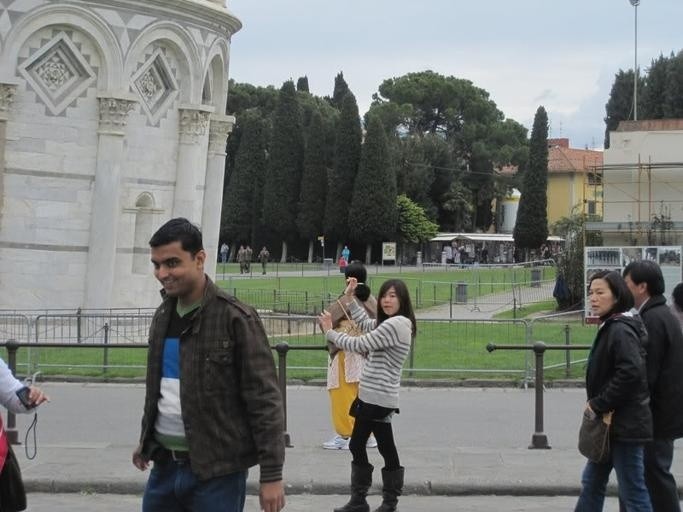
[165,446,188,459]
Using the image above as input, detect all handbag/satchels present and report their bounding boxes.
[576,406,616,463]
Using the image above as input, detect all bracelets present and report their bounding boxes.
[587,403,595,415]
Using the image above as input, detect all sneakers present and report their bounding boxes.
[367,437,377,450]
[324,436,351,452]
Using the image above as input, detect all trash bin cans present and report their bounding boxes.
[456,283,468,305]
[531,269,541,287]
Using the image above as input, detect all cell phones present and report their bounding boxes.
[17,387,36,409]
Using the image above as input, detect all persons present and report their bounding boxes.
[219,242,230,263]
[236,244,248,275]
[130,215,293,512]
[340,245,350,265]
[572,269,656,512]
[318,261,377,451]
[257,245,270,275]
[314,275,416,512]
[0,355,51,511]
[621,259,681,512]
[244,245,254,273]
[665,283,681,334]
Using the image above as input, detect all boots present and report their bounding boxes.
[375,466,405,512]
[334,461,375,511]
